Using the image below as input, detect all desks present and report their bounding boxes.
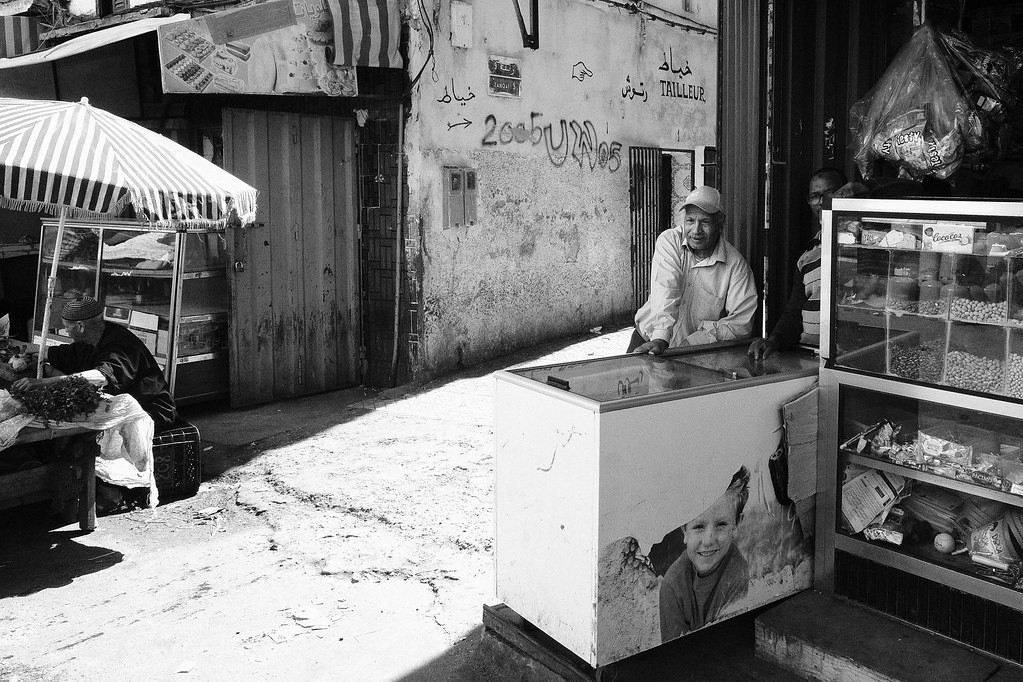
[0,401,107,532]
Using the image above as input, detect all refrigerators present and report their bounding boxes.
[494,338,813,670]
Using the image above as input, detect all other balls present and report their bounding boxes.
[933,532,955,554]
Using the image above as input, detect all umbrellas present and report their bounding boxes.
[0,97,261,378]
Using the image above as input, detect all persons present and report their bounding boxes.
[635,349,781,394]
[626,186,758,354]
[747,168,879,359]
[659,466,751,642]
[13,296,176,515]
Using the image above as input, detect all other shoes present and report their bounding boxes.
[109,493,150,515]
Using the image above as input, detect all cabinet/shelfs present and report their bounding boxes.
[29,217,224,403]
[816,197,1023,669]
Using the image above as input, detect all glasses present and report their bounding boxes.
[807,190,835,206]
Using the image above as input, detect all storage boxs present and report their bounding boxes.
[917,424,1002,469]
[137,420,201,507]
[104,290,226,358]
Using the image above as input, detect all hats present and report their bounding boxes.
[60,296,103,321]
[678,186,726,216]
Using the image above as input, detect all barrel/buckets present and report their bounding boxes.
[966,510,1023,566]
